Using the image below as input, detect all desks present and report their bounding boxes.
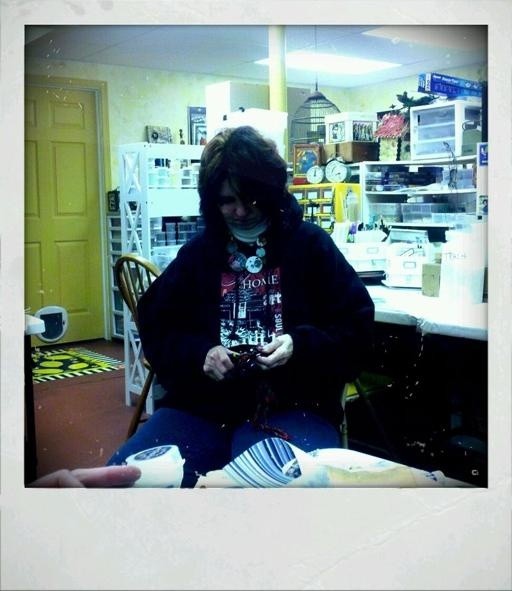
[25,312,45,483]
[363,282,488,343]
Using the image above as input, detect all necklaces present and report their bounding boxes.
[219,233,272,274]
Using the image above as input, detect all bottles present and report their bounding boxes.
[344,187,358,224]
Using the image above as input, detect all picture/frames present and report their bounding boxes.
[293,143,322,184]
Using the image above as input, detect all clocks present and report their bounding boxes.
[307,156,348,183]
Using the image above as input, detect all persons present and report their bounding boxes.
[26,465,141,486]
[101,126,375,488]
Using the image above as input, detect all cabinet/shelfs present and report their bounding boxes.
[288,182,361,236]
[108,214,204,341]
[362,154,478,228]
[119,143,204,413]
[410,99,483,158]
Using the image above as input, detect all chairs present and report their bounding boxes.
[115,253,164,441]
[340,378,350,449]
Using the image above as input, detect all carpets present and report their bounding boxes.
[33,346,133,384]
